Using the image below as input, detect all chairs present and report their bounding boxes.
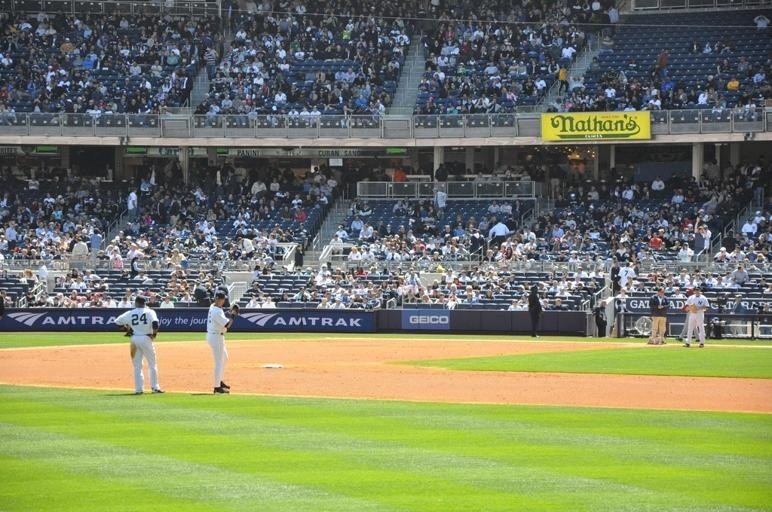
[586,18,770,93]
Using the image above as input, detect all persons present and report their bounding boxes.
[114,296,165,395]
[207,290,239,393]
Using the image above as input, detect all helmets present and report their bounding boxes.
[135,295,146,303]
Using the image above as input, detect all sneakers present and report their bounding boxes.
[213,381,230,393]
[151,387,164,393]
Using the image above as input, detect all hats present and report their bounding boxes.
[214,290,226,297]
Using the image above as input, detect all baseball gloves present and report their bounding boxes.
[124,328,133,336]
[230,304,239,317]
